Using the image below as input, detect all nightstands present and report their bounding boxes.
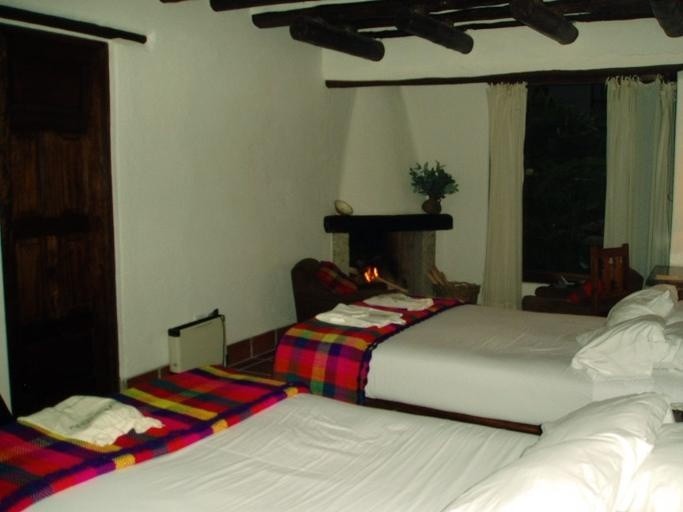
[645,264,683,302]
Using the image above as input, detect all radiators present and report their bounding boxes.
[168,309,228,374]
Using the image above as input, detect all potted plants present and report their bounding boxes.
[409,160,459,213]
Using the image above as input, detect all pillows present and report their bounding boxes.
[653,321,683,372]
[313,261,360,300]
[616,421,683,512]
[521,392,676,482]
[664,299,683,326]
[604,284,678,329]
[567,265,623,304]
[570,314,668,382]
[439,438,623,512]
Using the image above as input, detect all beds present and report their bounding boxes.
[273,290,683,436]
[0,363,682,512]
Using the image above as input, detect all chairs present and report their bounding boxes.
[522,268,644,317]
[291,257,388,323]
[590,243,636,316]
